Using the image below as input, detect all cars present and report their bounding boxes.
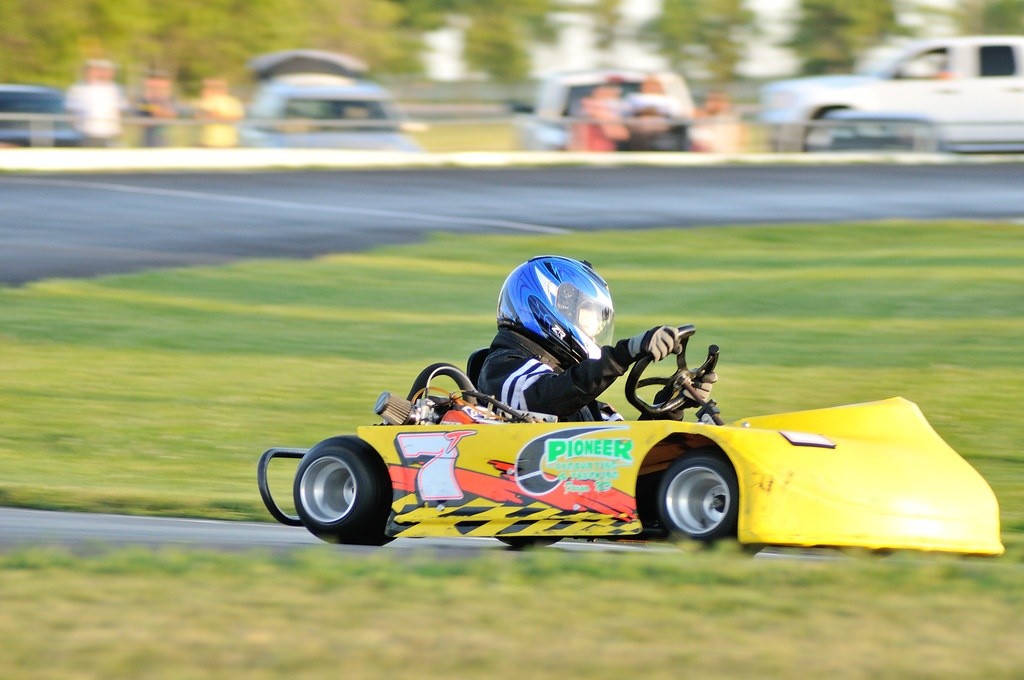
[507,60,701,154]
[238,51,419,153]
[0,83,83,148]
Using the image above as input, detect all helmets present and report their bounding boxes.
[497,255,614,370]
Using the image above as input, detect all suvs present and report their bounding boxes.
[754,35,1024,153]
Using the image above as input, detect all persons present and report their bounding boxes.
[189,76,243,149]
[570,72,743,151]
[64,58,132,148]
[477,257,718,424]
[134,69,195,147]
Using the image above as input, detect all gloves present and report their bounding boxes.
[614,325,682,363]
[667,368,718,413]
